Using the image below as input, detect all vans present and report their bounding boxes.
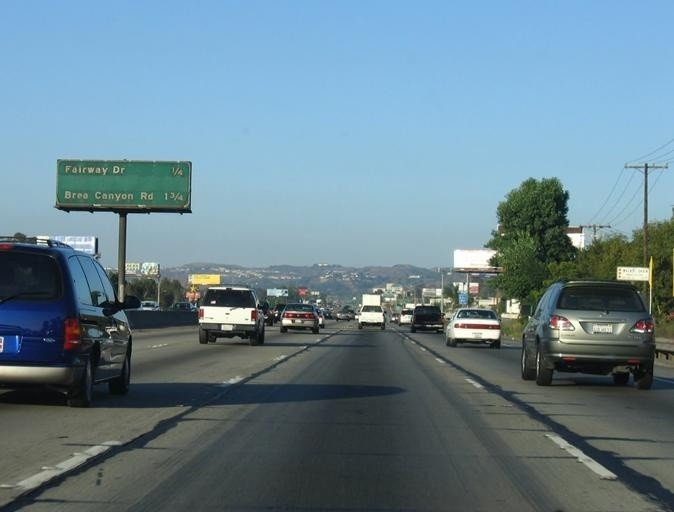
[0,234,141,408]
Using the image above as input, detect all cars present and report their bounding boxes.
[389,312,401,324]
[137,298,197,312]
[445,308,502,349]
[259,300,356,328]
[279,303,319,334]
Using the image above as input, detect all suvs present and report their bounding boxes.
[198,285,269,345]
[521,280,655,390]
[398,309,414,327]
[410,305,446,335]
[358,305,388,330]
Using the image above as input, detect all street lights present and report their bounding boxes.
[439,271,453,314]
[459,268,469,311]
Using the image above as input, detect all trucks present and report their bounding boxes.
[358,293,381,308]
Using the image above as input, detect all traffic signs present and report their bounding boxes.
[57,158,189,209]
